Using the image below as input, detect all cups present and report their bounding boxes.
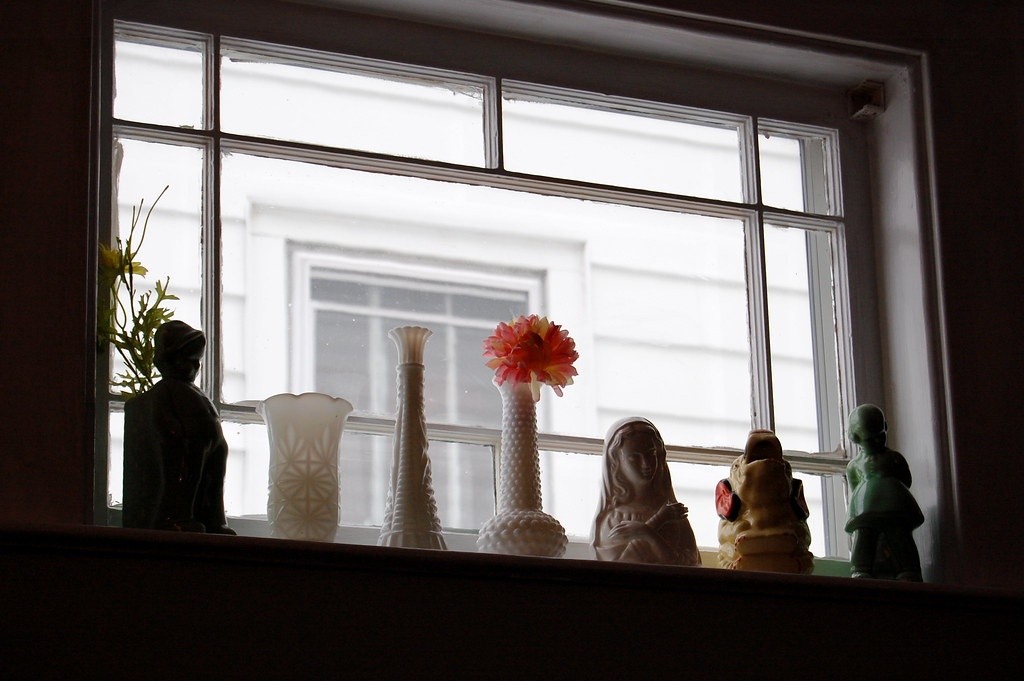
[255,392,354,541]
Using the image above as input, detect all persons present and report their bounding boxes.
[847,403,925,582]
[122,320,234,534]
[591,417,703,567]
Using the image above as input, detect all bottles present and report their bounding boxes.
[374,326,452,551]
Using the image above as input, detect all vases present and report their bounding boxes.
[376,325,448,550]
[477,375,570,558]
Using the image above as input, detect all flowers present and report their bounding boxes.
[486,313,579,400]
